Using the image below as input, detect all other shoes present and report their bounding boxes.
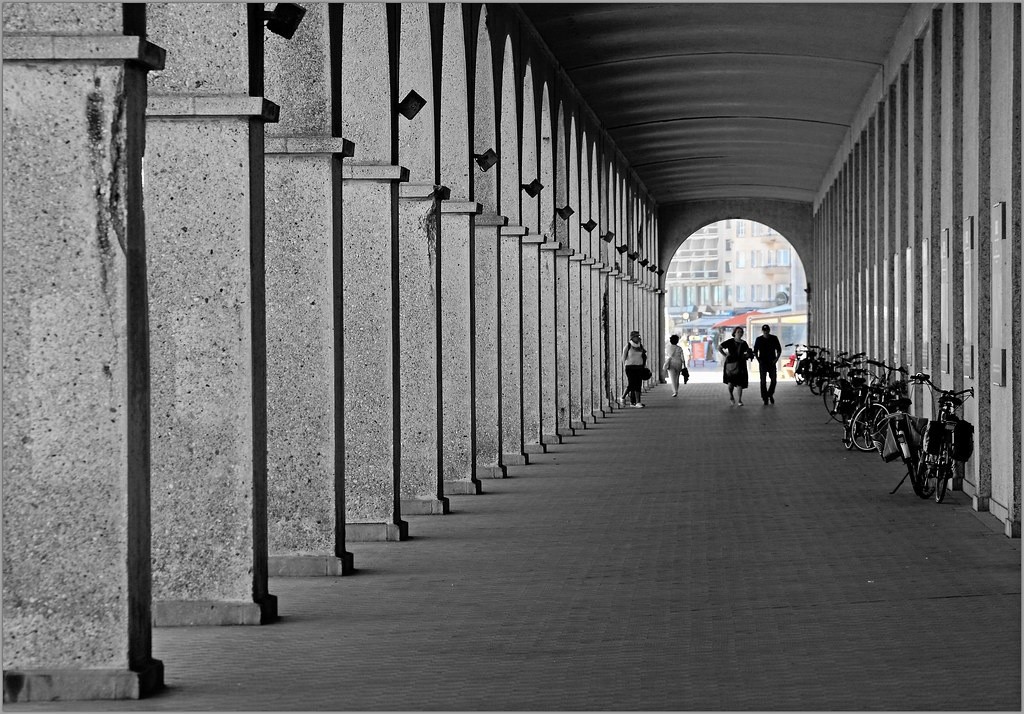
[763,398,768,405]
[630,403,636,407]
[620,396,626,407]
[738,402,743,406]
[636,403,644,408]
[672,393,677,397]
[770,396,774,404]
[729,399,735,405]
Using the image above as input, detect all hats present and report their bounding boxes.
[762,325,770,330]
[630,331,640,338]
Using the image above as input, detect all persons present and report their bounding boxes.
[619,331,647,408]
[754,325,782,405]
[718,326,750,406]
[666,335,689,396]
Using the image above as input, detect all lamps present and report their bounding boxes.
[265,3,308,40]
[581,219,597,233]
[638,259,649,267]
[399,89,427,120]
[616,244,628,255]
[556,204,575,222]
[655,269,664,277]
[474,147,500,173]
[522,178,544,198]
[647,265,657,272]
[628,252,639,261]
[600,230,614,243]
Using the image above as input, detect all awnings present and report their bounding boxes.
[677,312,764,343]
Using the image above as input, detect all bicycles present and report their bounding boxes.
[911,372,975,504]
[865,358,923,497]
[784,342,892,453]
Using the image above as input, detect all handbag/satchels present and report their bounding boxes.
[906,413,929,450]
[923,420,945,455]
[952,420,974,461]
[663,357,672,370]
[726,362,739,376]
[642,368,652,380]
[870,417,901,463]
